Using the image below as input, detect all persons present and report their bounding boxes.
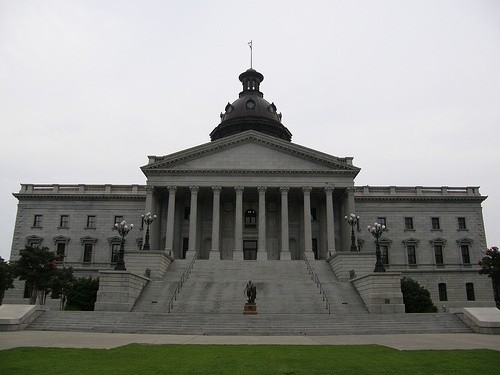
[244,280,254,300]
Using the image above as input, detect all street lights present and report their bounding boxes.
[367,222,387,272]
[344,213,360,252]
[115,220,134,270]
[141,211,157,250]
